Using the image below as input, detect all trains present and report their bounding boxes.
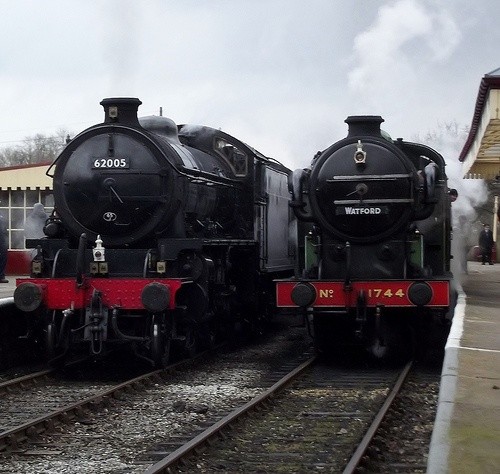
[274,113,463,362]
[13,95,299,367]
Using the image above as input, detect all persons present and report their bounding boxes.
[478,224,494,265]
[0,215,9,283]
[458,215,477,274]
